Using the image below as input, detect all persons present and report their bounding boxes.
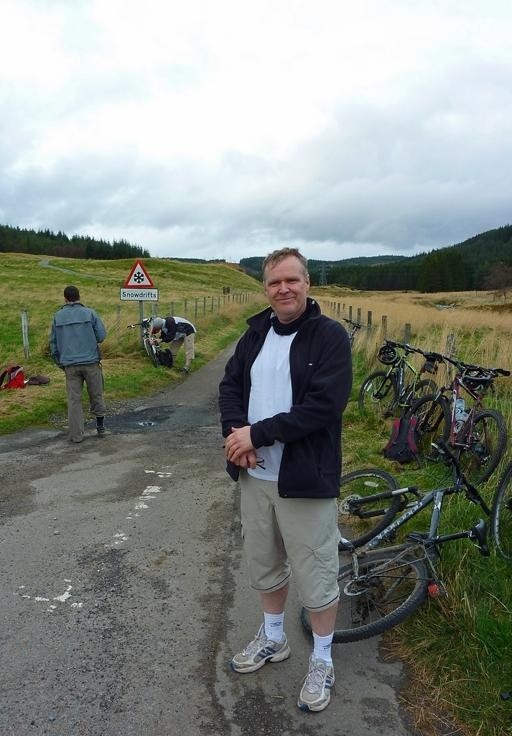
[219,248,352,712]
[150,317,196,372]
[50,286,106,435]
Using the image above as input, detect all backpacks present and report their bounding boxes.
[381,416,422,465]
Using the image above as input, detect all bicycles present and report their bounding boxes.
[339,315,362,350]
[125,316,162,369]
[356,335,441,435]
[297,439,496,648]
[398,351,512,492]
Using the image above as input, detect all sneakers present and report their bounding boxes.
[297,652,335,712]
[180,366,191,373]
[231,623,291,674]
[95,423,106,434]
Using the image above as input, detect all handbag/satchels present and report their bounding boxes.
[157,348,173,368]
[0,364,50,389]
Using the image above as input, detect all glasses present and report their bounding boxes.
[256,457,266,470]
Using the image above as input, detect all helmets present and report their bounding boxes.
[377,345,401,365]
